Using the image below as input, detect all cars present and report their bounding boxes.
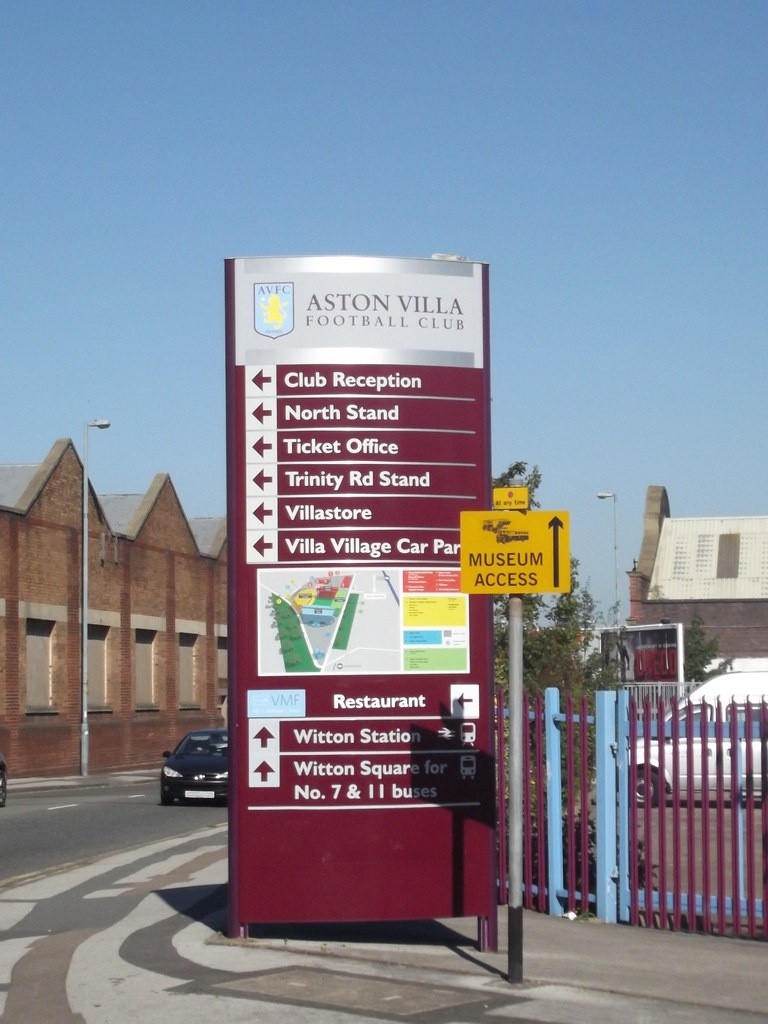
[0,753,7,807]
[159,728,230,804]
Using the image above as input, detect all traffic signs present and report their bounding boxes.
[241,466,492,500]
[235,751,501,809]
[242,364,486,400]
[239,431,488,468]
[236,718,497,752]
[460,510,573,596]
[242,501,492,530]
[240,399,488,432]
[241,529,460,564]
[237,675,486,720]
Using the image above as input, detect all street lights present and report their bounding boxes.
[597,492,619,627]
[79,419,113,774]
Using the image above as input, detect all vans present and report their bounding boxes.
[629,657,768,808]
